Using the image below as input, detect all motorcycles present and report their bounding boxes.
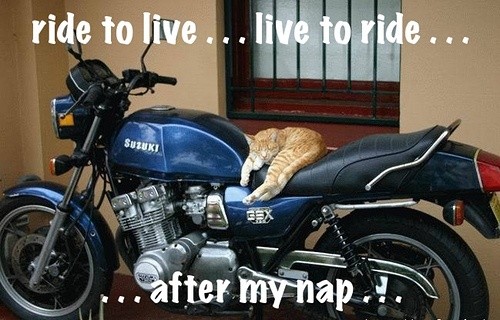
[0,19,500,320]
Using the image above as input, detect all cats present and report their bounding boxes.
[239,126,329,206]
